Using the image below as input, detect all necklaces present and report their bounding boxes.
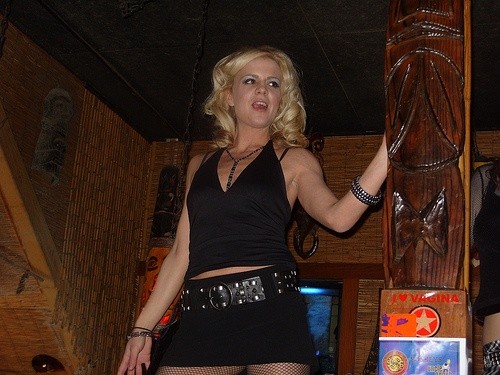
[224,145,266,193]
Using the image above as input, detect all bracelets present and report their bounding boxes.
[126,327,153,340]
[350,174,382,206]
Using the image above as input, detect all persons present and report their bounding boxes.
[472,159,500,375]
[116,45,389,375]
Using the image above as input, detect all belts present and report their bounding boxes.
[183,268,301,311]
